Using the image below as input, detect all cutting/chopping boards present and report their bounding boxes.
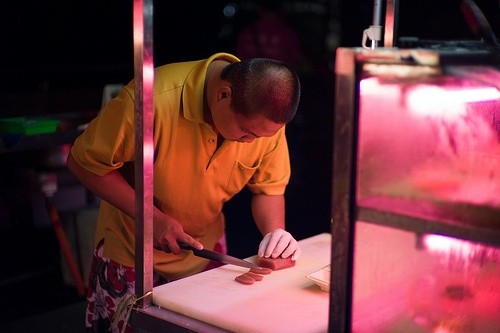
[151,230,472,333]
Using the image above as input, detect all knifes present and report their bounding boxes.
[176,241,263,270]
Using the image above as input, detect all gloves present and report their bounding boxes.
[258,228,301,261]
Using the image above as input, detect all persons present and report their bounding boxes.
[66,53,301,333]
